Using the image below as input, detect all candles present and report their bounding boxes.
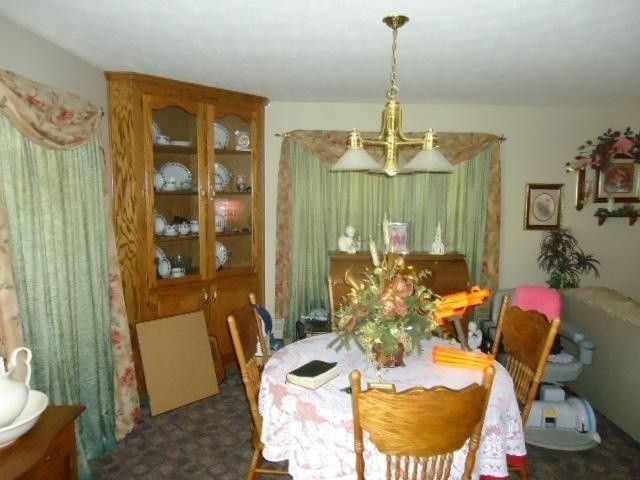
[607,198,614,212]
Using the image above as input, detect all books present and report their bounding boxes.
[285,359,339,389]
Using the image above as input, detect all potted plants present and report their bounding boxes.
[536,226,601,288]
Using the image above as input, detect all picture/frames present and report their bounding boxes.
[524,184,563,230]
[592,154,640,203]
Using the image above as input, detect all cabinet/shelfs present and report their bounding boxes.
[0,405,86,480]
[104,71,269,402]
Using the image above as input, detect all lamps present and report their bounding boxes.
[328,15,456,176]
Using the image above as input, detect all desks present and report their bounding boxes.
[326,251,469,344]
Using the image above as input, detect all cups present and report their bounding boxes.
[172,267,186,278]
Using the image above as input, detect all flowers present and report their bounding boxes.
[565,127,640,177]
[331,253,446,368]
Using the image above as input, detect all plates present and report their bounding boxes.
[159,162,192,187]
[171,140,192,147]
[216,241,228,270]
[155,246,172,279]
[215,162,231,192]
[151,212,167,235]
[214,122,229,150]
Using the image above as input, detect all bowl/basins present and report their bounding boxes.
[165,220,199,237]
[0,390,49,449]
